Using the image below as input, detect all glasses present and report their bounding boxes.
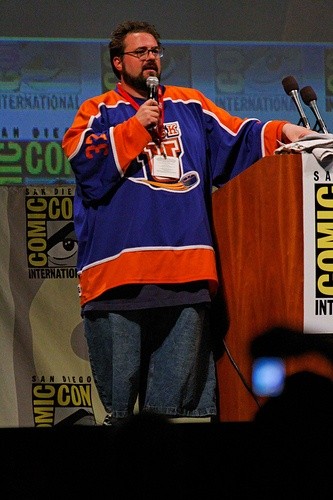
[119,48,164,58]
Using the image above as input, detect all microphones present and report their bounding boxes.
[282,76,311,133]
[298,84,328,135]
[146,75,159,131]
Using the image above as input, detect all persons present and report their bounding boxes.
[62,16,318,429]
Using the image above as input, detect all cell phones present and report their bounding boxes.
[249,356,284,396]
